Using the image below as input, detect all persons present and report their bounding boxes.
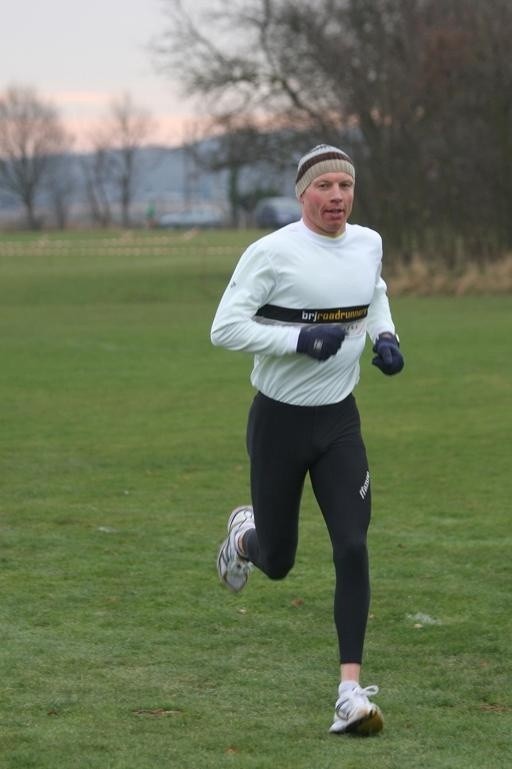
[208,144,406,738]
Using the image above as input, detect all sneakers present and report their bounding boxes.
[217,506,256,594]
[329,685,383,734]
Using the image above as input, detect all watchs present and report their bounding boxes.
[379,332,400,348]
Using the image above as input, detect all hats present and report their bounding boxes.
[295,144,355,200]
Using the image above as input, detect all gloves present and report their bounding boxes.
[296,325,345,361]
[373,332,403,374]
